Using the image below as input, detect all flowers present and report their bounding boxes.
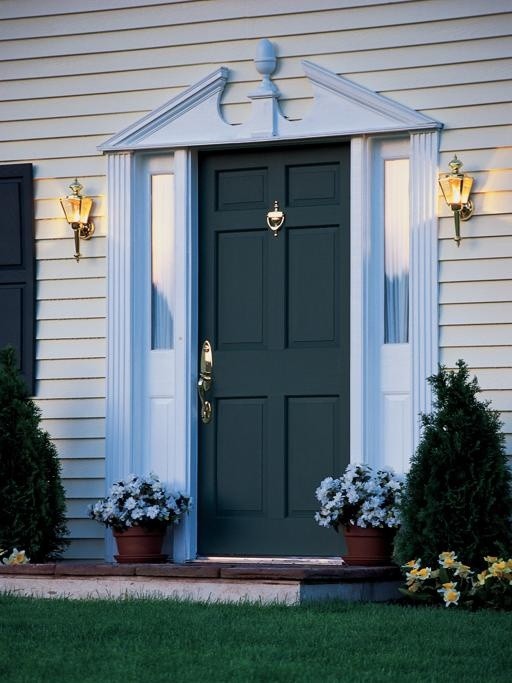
[313,461,405,529]
[86,472,193,530]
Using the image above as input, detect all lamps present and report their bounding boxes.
[59,177,95,264]
[436,154,474,247]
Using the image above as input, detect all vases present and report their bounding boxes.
[112,522,168,555]
[341,524,401,557]
